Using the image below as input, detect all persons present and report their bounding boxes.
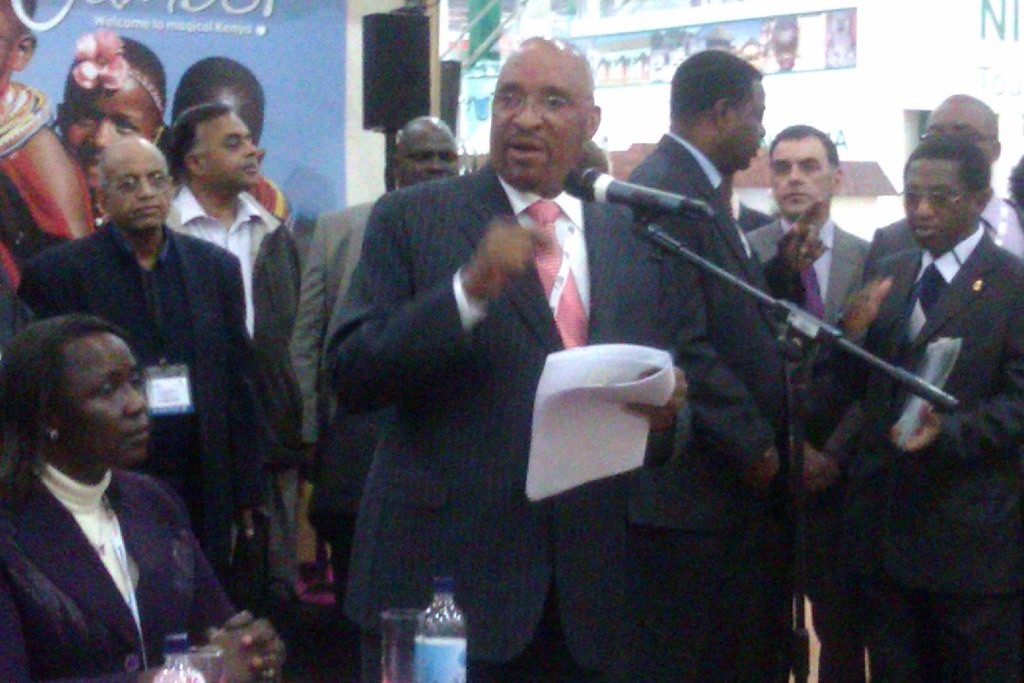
[3,135,254,597]
[773,20,798,70]
[0,314,287,683]
[162,104,303,616]
[289,116,459,683]
[0,0,291,310]
[621,51,827,683]
[732,94,1024,683]
[328,39,688,683]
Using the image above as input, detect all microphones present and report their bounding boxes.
[563,167,714,221]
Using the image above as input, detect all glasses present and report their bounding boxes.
[489,91,595,117]
[919,132,994,148]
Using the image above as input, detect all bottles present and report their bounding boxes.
[150,633,206,683]
[414,574,468,683]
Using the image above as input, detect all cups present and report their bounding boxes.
[378,607,423,683]
[187,645,228,683]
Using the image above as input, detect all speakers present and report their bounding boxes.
[362,13,431,133]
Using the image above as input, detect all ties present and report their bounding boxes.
[801,262,824,321]
[525,198,590,350]
[918,265,940,317]
